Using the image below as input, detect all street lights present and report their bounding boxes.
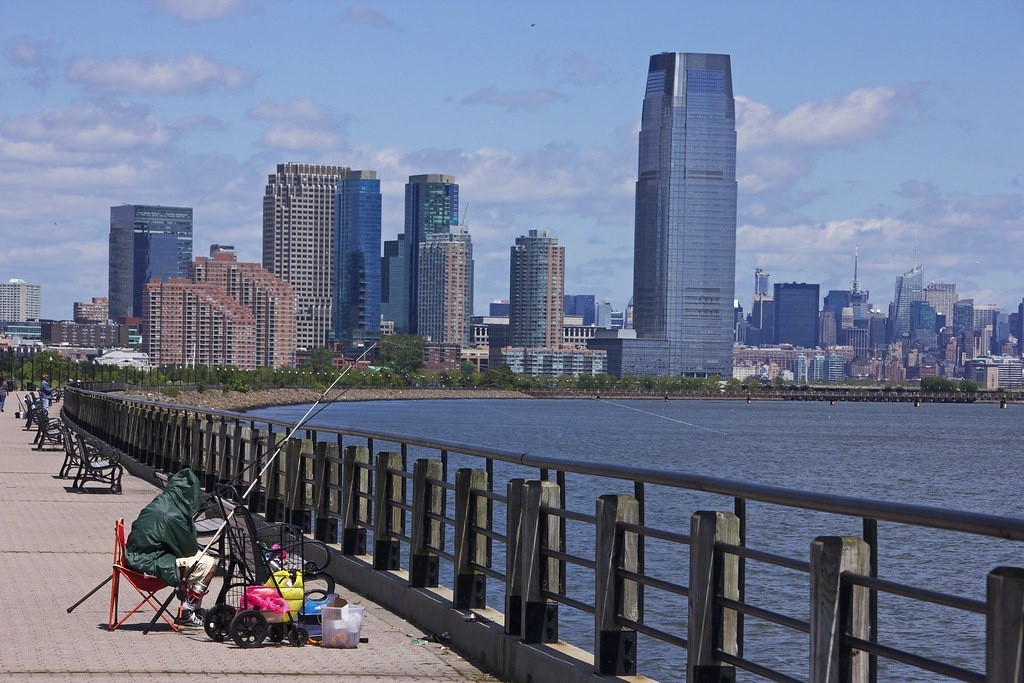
[179,365,182,385]
[141,363,143,386]
[566,378,609,392]
[316,372,342,384]
[164,364,167,387]
[302,371,305,384]
[363,372,382,387]
[309,371,312,386]
[91,360,96,382]
[431,375,469,390]
[48,356,54,389]
[288,370,291,386]
[347,373,350,383]
[149,364,153,377]
[216,367,220,380]
[75,359,78,382]
[224,368,249,378]
[296,371,298,385]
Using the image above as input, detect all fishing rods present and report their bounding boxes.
[142,342,381,634]
[65,368,376,614]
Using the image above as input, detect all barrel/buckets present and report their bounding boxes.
[15,412,25,419]
[321,604,366,648]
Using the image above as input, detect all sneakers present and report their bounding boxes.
[174,608,206,630]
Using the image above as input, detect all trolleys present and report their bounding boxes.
[205,482,309,649]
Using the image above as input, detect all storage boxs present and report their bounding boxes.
[319,604,365,649]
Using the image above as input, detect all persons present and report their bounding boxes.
[0,376,9,412]
[39,374,57,417]
[124,468,216,629]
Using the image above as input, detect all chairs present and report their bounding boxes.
[108,517,186,633]
[72,434,125,495]
[58,425,104,481]
[25,388,75,452]
